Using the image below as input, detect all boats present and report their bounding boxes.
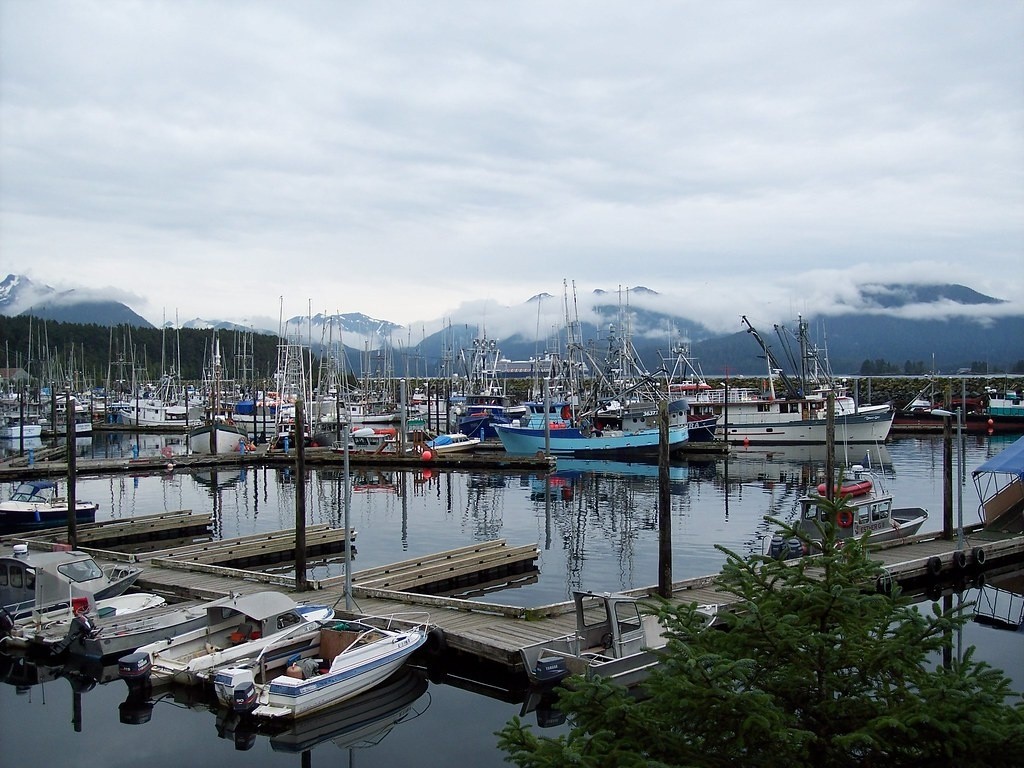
[1,591,164,649]
[713,441,897,481]
[766,462,932,573]
[193,611,432,721]
[527,455,694,503]
[117,591,338,697]
[0,480,102,529]
[215,657,432,768]
[64,587,243,661]
[0,543,144,626]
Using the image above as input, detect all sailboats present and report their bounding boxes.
[0,274,1023,464]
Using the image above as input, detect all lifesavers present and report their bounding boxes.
[951,577,968,594]
[971,547,986,566]
[952,551,967,570]
[836,510,854,528]
[927,556,943,576]
[927,582,943,602]
[972,572,986,590]
